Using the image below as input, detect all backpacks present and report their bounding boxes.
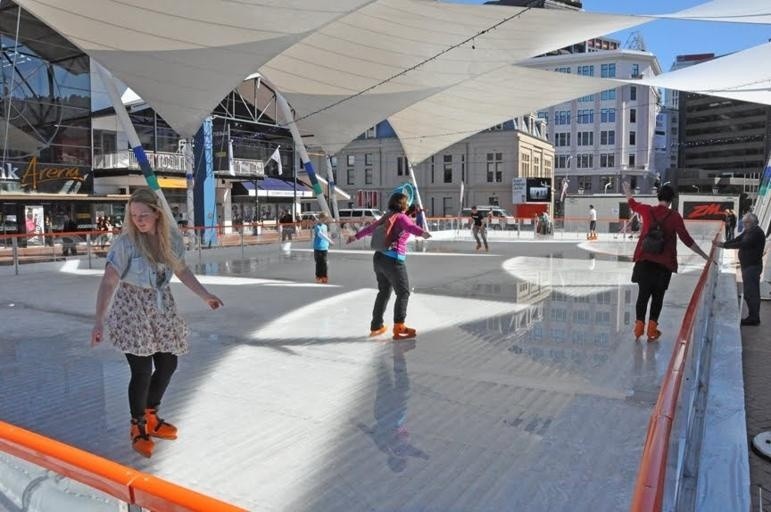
[370,211,401,252]
[640,206,675,255]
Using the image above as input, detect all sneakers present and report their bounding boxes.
[740,317,761,326]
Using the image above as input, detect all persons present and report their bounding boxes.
[56,213,79,262]
[231,205,242,219]
[91,186,224,455]
[280,209,294,240]
[312,212,336,283]
[727,209,738,240]
[44,215,54,247]
[623,346,662,411]
[588,205,598,239]
[345,193,433,333]
[723,208,731,240]
[471,206,490,250]
[350,345,431,473]
[25,208,43,246]
[713,212,766,326]
[629,209,642,238]
[621,181,718,338]
[94,214,123,250]
[535,208,553,236]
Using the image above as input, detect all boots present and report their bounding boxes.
[370,322,388,332]
[475,244,489,251]
[646,319,662,337]
[130,409,177,453]
[315,276,328,284]
[586,235,598,239]
[393,323,416,335]
[633,319,645,337]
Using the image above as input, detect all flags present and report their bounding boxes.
[271,148,284,176]
